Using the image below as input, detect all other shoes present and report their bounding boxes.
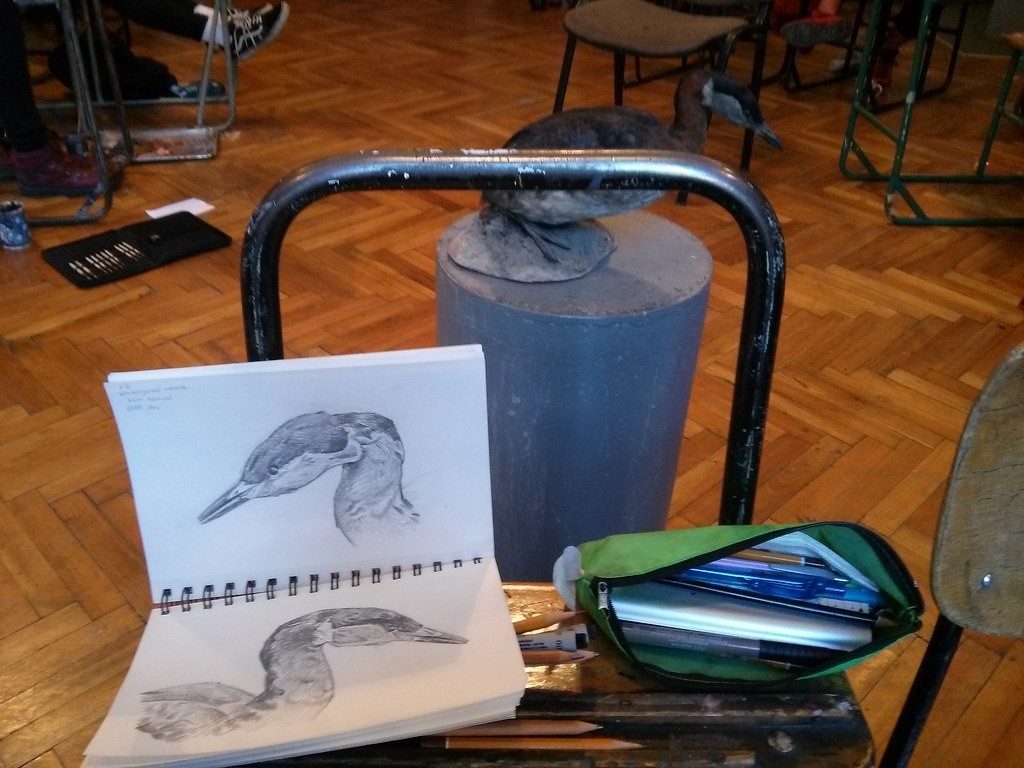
[0,201,31,251]
[15,144,125,197]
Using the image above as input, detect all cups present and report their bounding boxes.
[0,200,32,251]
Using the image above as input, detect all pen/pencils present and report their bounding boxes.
[616,548,895,675]
[416,607,652,751]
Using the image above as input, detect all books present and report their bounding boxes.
[82,343,526,768]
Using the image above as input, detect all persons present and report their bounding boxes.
[782,0,930,99]
[0,0,290,199]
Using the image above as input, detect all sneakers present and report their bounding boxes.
[217,1,289,60]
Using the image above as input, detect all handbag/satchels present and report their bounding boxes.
[570,521,925,683]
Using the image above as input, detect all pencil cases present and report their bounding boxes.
[571,520,927,689]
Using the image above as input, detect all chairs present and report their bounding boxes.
[554,0,773,206]
